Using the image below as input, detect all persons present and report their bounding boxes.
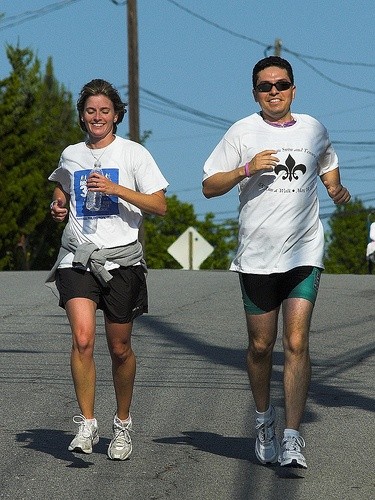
[201,56,351,468]
[49,79,169,459]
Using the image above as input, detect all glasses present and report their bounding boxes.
[254,82,292,92]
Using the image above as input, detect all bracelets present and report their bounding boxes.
[244,163,251,178]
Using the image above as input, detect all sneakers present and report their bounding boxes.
[68,413,99,454]
[278,436,308,469]
[107,417,134,460]
[255,406,280,464]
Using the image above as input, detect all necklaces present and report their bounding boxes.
[88,142,109,160]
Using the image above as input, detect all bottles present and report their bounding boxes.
[86,161,103,212]
